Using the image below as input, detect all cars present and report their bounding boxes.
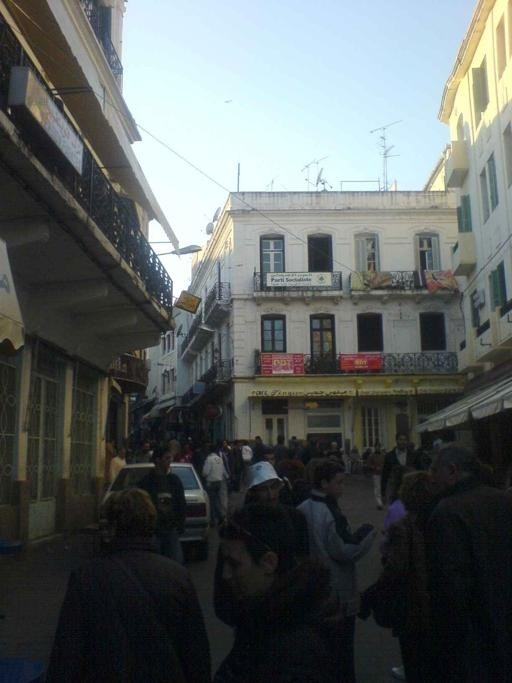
[99,461,210,561]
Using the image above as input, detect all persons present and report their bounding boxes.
[379,432,421,503]
[370,469,442,683]
[296,459,380,682]
[43,484,211,683]
[379,464,414,679]
[210,460,309,631]
[420,439,510,682]
[139,444,189,569]
[103,416,389,516]
[207,503,348,681]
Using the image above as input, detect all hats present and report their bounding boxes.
[245,460,286,497]
